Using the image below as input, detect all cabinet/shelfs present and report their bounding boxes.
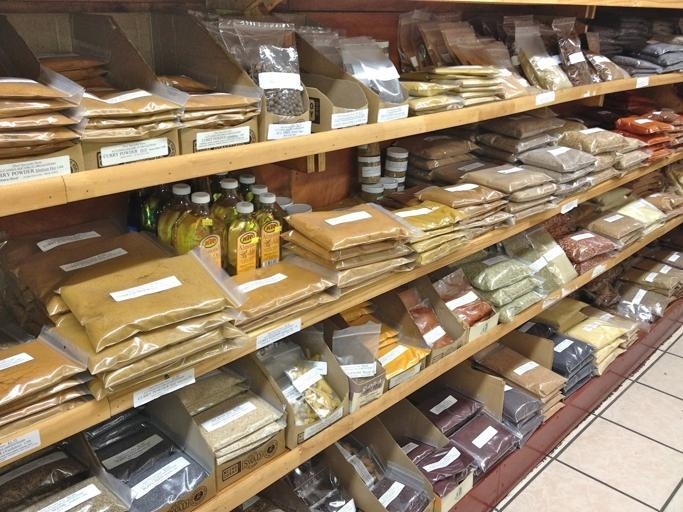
[0,2,683,512]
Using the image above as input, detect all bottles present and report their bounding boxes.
[124,172,284,281]
[356,142,410,206]
